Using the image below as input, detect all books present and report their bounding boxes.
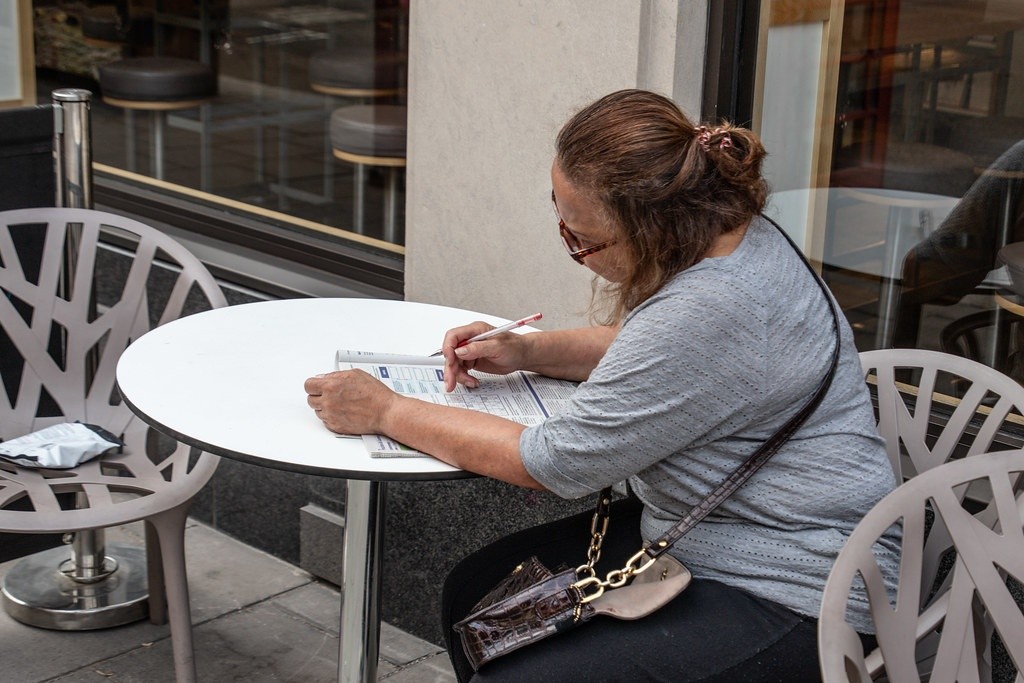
[334,349,590,459]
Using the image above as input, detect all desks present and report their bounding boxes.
[894,10,1021,154]
[764,187,1012,348]
[116,297,578,683]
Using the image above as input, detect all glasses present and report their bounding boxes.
[552,188,636,265]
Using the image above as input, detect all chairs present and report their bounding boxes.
[0,207,232,631]
[815,349,1024,683]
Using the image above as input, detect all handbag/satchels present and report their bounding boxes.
[452,550,692,672]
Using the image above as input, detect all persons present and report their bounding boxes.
[301,86,905,683]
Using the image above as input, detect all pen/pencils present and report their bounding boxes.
[428,312,542,357]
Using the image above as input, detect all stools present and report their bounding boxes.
[853,143,974,195]
[989,241,1024,378]
[310,47,407,246]
[103,56,217,181]
